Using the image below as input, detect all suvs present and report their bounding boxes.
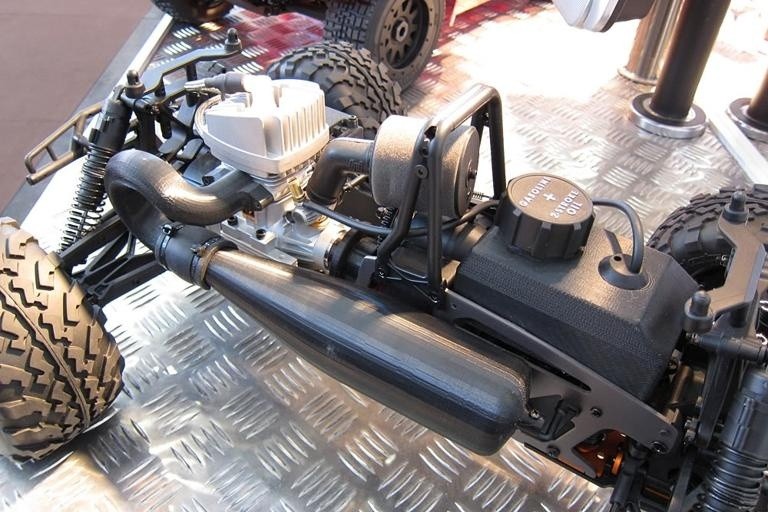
[1,28,766,511]
[150,1,446,97]
